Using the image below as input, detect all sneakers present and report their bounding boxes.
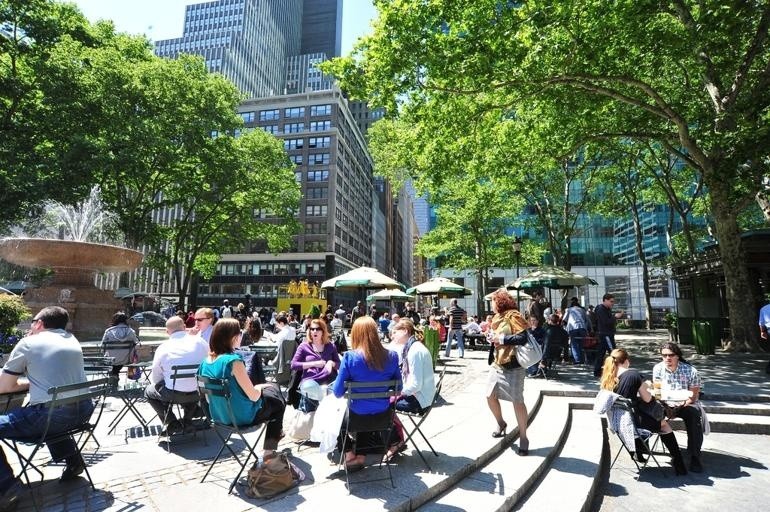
[690,458,703,474]
[528,372,541,377]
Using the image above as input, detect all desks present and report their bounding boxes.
[0,368,58,493]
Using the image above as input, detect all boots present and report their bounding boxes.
[635,437,647,463]
[660,432,688,476]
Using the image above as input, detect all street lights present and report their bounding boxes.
[511,236,523,312]
[153,274,169,314]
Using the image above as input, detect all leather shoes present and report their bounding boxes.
[159,417,210,436]
[59,459,84,482]
[0,480,25,509]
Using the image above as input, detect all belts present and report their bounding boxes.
[32,399,91,410]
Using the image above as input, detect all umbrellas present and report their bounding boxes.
[405,276,475,309]
[365,288,416,303]
[320,263,407,315]
[503,264,599,314]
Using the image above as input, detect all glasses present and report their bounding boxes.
[310,327,321,331]
[194,318,210,322]
[662,353,675,357]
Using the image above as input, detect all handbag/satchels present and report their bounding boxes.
[515,331,543,368]
[583,330,599,350]
[288,392,316,441]
[127,356,141,380]
[637,396,664,421]
[244,452,301,499]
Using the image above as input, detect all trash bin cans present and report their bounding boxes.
[691,320,717,354]
[144,316,152,327]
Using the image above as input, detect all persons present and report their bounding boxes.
[758,304,770,375]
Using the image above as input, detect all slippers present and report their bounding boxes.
[339,457,357,472]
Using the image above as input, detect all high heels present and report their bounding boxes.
[519,438,530,456]
[492,422,507,437]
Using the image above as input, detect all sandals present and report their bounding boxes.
[381,440,407,462]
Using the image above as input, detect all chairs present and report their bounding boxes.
[385,367,446,472]
[445,317,601,379]
[79,355,117,453]
[0,376,119,512]
[607,397,685,480]
[196,373,274,496]
[97,317,451,455]
[337,379,399,496]
